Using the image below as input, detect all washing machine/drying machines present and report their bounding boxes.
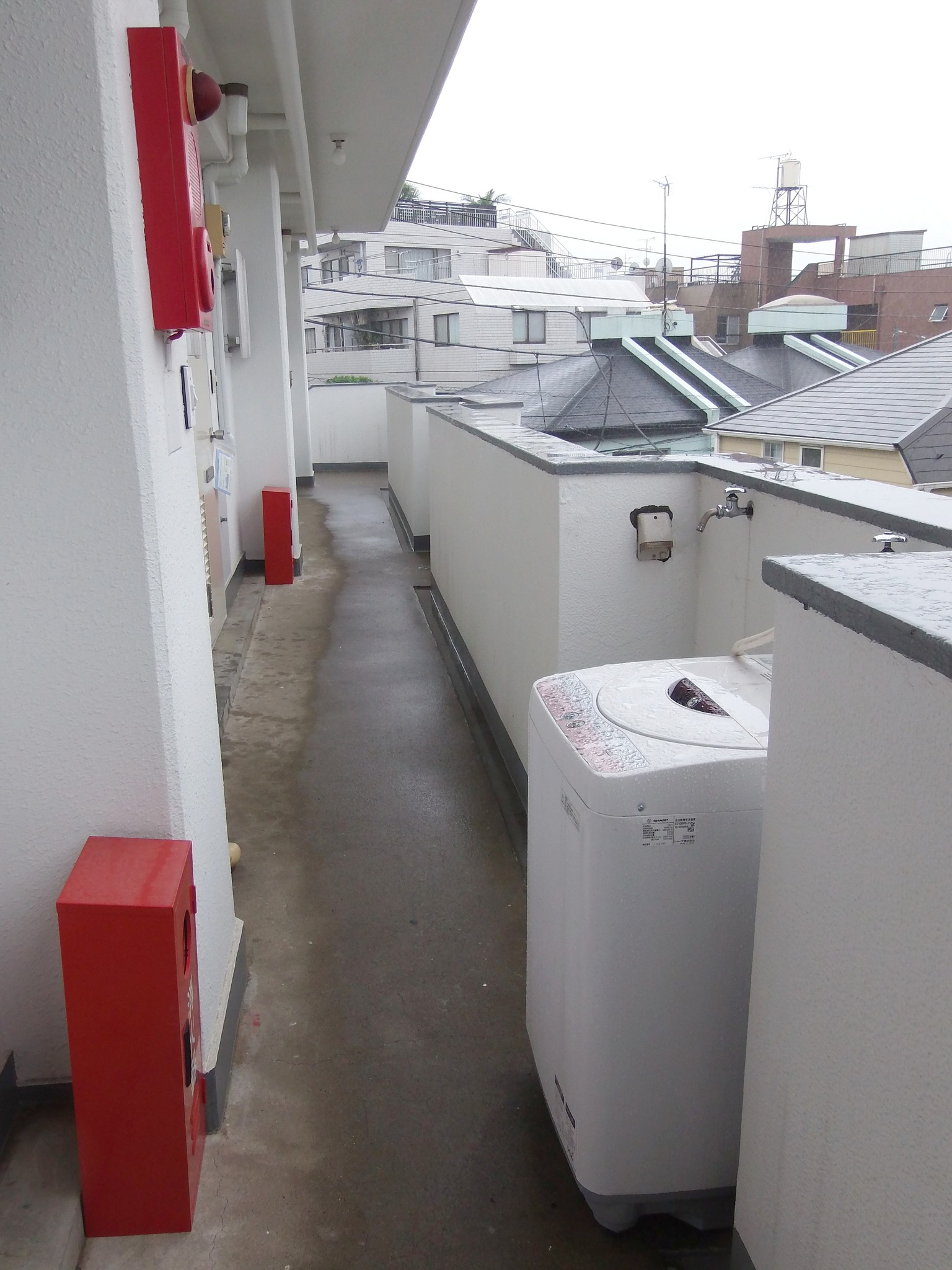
[523,654,772,1227]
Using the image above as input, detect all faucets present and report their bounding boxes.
[699,485,755,532]
